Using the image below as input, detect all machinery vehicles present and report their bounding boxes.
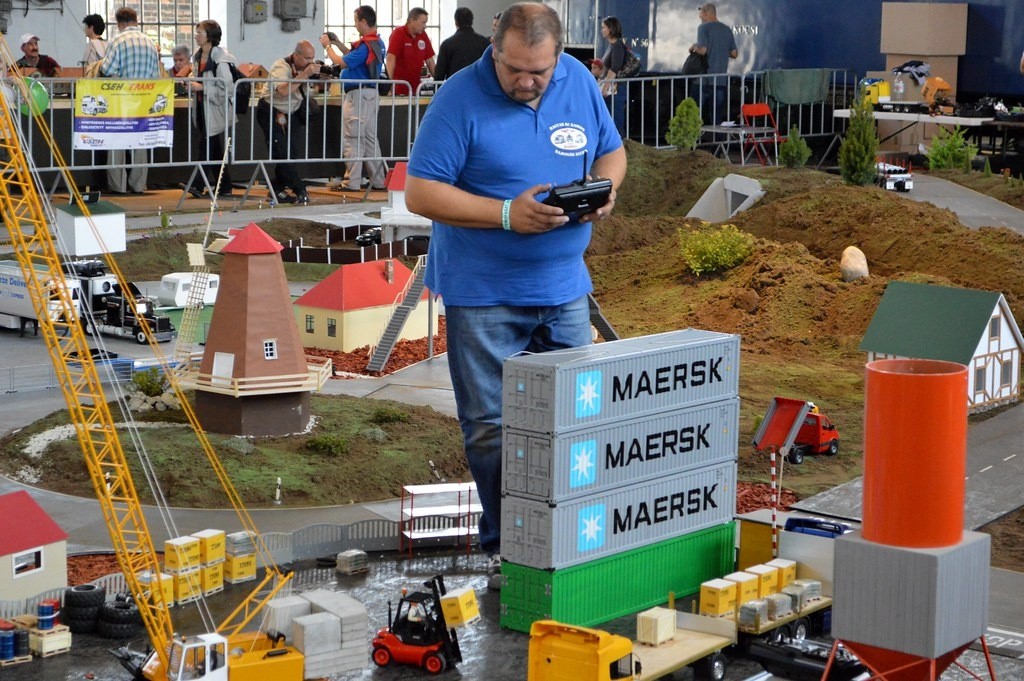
[369,575,482,674]
[0,28,304,681]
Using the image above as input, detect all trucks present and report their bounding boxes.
[750,397,841,464]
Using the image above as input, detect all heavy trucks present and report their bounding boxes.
[0,257,178,345]
[853,76,930,112]
[873,155,913,193]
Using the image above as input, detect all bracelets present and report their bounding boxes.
[325,45,331,51]
[502,199,512,232]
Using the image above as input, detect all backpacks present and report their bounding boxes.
[211,61,251,114]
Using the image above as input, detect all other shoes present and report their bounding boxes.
[206,190,232,198]
[179,183,202,197]
[329,184,359,192]
[361,182,386,191]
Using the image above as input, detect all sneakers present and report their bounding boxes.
[296,191,310,202]
[487,552,501,590]
[267,191,298,204]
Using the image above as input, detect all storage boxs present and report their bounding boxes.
[498,326,797,639]
[866,2,969,153]
[153,528,257,611]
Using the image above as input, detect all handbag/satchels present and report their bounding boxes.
[681,51,709,80]
[295,98,322,125]
[378,76,392,96]
[611,40,642,78]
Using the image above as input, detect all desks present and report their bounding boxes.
[8,96,434,194]
[699,126,776,167]
[817,109,994,169]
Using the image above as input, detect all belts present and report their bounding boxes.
[345,86,375,94]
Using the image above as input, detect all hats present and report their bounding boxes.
[19,33,40,48]
[589,59,602,66]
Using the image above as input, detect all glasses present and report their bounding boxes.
[698,7,705,12]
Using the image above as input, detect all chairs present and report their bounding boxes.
[743,102,788,163]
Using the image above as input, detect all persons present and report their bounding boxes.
[590,15,628,140]
[319,4,386,191]
[256,40,327,203]
[308,59,341,93]
[6,32,62,78]
[385,7,436,96]
[81,6,236,200]
[432,7,504,94]
[688,3,739,157]
[403,2,628,591]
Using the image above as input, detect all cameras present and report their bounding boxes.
[310,64,341,80]
[322,32,335,40]
